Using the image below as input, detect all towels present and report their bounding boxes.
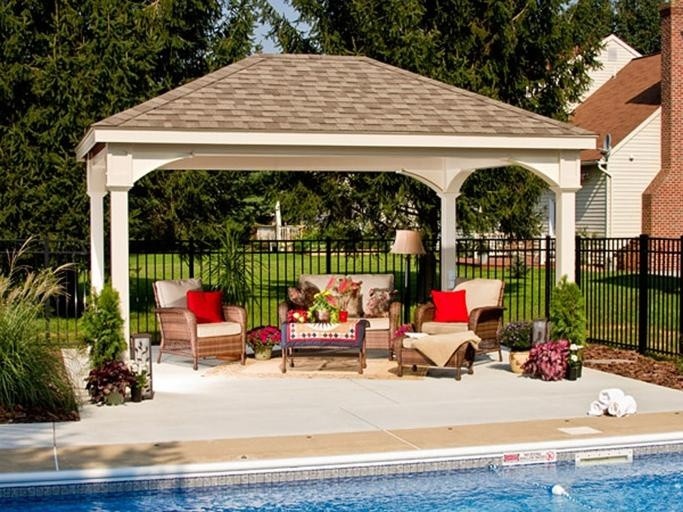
[609,398,638,417]
[586,400,609,418]
[599,389,623,402]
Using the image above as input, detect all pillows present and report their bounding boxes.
[430,289,470,324]
[286,285,302,306]
[185,290,224,323]
[303,281,319,306]
[319,275,362,310]
[365,288,397,318]
[347,289,361,317]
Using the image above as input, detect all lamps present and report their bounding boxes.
[390,230,426,322]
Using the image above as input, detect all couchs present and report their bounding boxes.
[279,273,402,361]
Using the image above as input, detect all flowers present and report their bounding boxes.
[494,320,532,351]
[308,289,341,321]
[245,326,281,350]
[564,342,579,367]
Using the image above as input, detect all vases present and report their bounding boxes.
[314,309,329,321]
[254,349,271,360]
[508,352,529,372]
[566,365,576,381]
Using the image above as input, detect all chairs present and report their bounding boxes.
[152,277,247,370]
[413,279,507,364]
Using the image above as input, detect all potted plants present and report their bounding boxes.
[128,368,148,402]
[548,275,588,382]
[85,359,137,404]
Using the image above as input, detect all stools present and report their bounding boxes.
[395,333,474,380]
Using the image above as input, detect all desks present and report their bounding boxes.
[280,320,369,374]
